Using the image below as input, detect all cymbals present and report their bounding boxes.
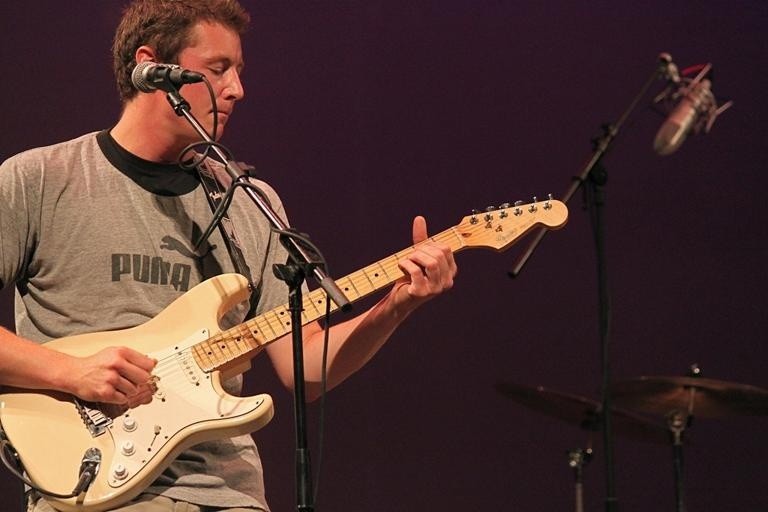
[596,376,768,417]
[495,384,666,445]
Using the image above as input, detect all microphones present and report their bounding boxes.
[132,60,206,92]
[655,79,714,164]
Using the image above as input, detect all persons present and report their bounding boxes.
[0,0,458,512]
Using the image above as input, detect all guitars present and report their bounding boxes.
[0,194,569,512]
